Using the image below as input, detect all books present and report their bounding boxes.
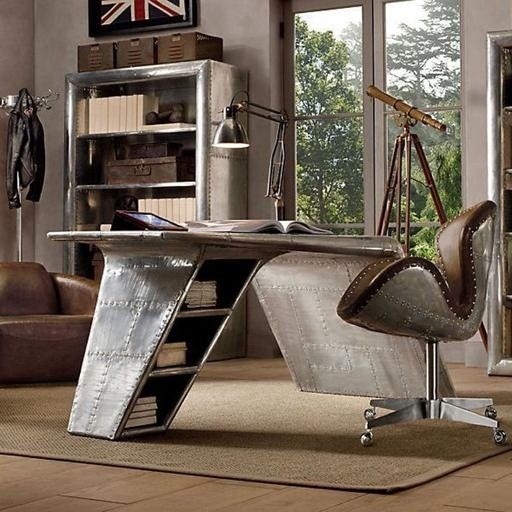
[137,198,196,226]
[185,219,335,235]
[123,280,219,430]
[76,94,196,134]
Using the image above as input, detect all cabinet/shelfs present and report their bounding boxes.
[61,58,249,362]
[484,27,511,376]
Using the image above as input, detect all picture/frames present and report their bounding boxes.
[88,0,197,37]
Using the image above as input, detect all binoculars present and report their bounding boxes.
[366,85,446,136]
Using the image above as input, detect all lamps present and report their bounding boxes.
[208,87,290,221]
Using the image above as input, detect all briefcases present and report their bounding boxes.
[104,143,178,184]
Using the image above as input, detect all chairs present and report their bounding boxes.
[335,200,506,449]
[0,259,99,387]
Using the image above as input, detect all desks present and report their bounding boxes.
[46,231,459,442]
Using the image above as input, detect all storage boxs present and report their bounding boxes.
[76,31,224,72]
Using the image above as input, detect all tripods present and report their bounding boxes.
[377,128,488,355]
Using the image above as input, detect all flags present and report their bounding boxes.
[100,0,184,25]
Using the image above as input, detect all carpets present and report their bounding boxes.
[0,355,511,494]
